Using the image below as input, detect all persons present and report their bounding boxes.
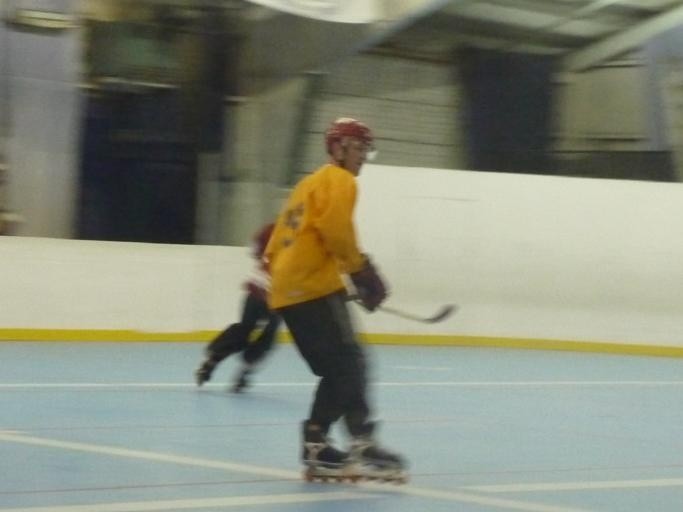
[259,117,402,471]
[195,221,283,390]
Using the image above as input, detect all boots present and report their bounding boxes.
[347,421,403,469]
[303,419,348,463]
[194,357,219,382]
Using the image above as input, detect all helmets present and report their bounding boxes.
[324,117,376,162]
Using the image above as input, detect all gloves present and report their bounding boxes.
[349,265,386,312]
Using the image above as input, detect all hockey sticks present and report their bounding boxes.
[355,297,455,324]
